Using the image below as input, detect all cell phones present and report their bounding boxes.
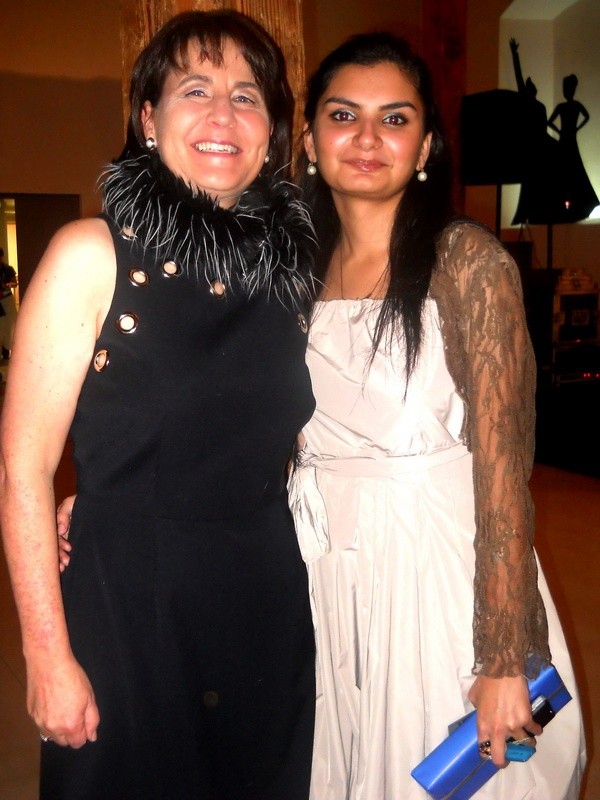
[505,695,556,744]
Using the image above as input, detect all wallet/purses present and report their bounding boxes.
[411,653,573,799]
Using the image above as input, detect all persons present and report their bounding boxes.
[53,32,586,800]
[0,248,18,358]
[0,8,331,800]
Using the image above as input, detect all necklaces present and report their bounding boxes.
[340,244,388,299]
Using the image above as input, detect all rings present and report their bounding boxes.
[41,735,49,742]
[478,740,491,755]
